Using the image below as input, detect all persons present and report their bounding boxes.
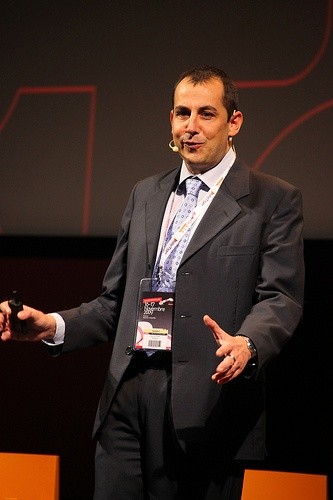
[1,67,305,500]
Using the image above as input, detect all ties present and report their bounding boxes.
[151,178,202,293]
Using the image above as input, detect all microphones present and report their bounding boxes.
[167,139,180,153]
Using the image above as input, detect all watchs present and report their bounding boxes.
[244,334,257,358]
